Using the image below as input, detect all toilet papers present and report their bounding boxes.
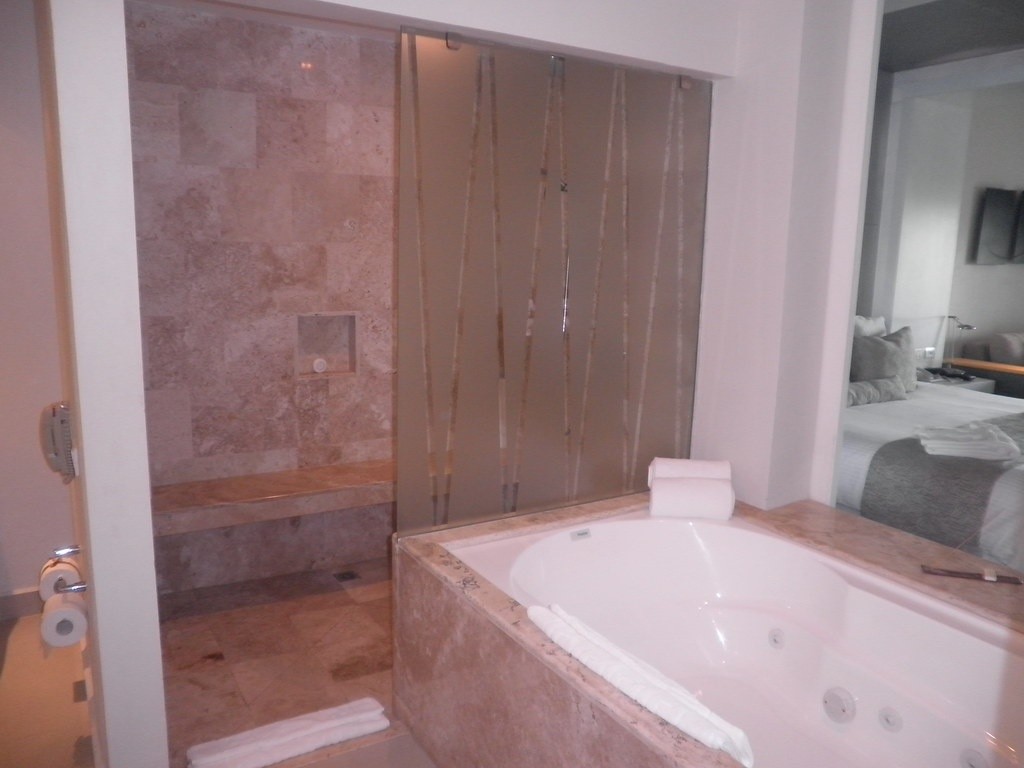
[37,554,85,606]
[37,592,90,660]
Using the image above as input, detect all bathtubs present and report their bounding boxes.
[438,508,1024,768]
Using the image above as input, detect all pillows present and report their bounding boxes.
[852,325,921,393]
[846,377,909,406]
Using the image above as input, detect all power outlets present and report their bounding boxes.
[925,347,936,359]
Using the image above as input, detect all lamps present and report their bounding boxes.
[943,315,977,377]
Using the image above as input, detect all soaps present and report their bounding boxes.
[982,567,999,582]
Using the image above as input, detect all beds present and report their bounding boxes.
[835,379,1023,576]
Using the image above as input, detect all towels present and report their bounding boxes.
[916,420,1022,462]
[186,696,391,768]
[647,477,737,523]
[644,457,735,494]
